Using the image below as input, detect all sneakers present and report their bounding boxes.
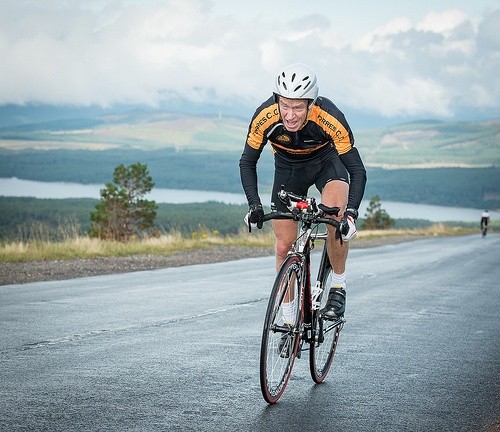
[279,323,296,358]
[323,286,346,316]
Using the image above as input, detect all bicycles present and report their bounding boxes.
[480,218,489,236]
[259,183,350,405]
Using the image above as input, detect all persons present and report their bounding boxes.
[240,63,367,358]
[481,210,490,231]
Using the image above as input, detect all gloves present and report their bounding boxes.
[244,205,263,229]
[335,208,359,242]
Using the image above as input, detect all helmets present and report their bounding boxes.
[273,63,318,110]
